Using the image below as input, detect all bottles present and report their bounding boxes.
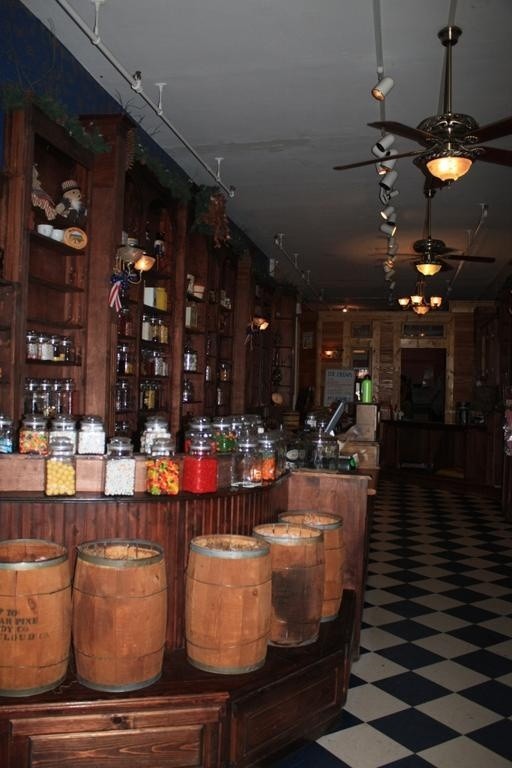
[145,439,181,494]
[185,413,266,450]
[361,373,372,403]
[182,434,220,492]
[26,330,77,360]
[272,323,284,366]
[181,337,232,405]
[24,378,79,414]
[260,430,290,481]
[77,415,106,454]
[103,436,135,496]
[115,309,170,411]
[1,413,13,454]
[139,416,173,456]
[232,435,263,485]
[107,419,134,456]
[18,416,47,456]
[44,437,76,496]
[49,416,78,455]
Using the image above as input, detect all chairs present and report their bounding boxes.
[284,404,387,663]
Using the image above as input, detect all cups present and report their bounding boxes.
[36,223,64,240]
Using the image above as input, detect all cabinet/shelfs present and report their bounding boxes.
[271,293,293,412]
[0,95,93,445]
[94,122,172,451]
[173,182,233,451]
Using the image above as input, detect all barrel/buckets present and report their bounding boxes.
[276,507,349,625]
[0,536,72,699]
[182,532,275,678]
[72,538,170,695]
[254,521,327,650]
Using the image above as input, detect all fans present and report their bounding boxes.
[331,26,511,171]
[381,188,498,264]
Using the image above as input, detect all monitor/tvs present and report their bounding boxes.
[324,400,345,434]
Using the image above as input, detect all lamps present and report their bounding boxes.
[413,259,446,276]
[369,70,399,275]
[398,278,443,317]
[243,316,269,344]
[413,144,485,184]
[112,248,155,284]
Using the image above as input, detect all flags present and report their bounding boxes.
[107,274,129,313]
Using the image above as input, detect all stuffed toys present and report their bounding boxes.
[27,162,87,234]
